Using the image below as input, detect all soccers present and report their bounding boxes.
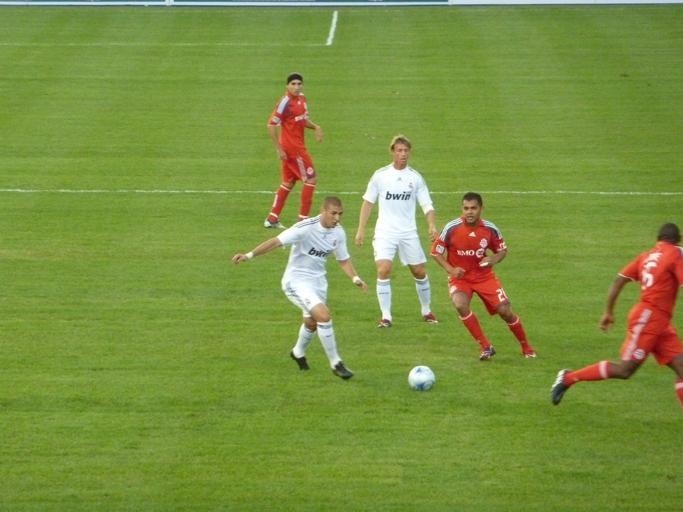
[409,366,435,391]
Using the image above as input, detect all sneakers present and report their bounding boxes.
[478,343,496,360]
[289,348,310,372]
[523,349,538,359]
[264,218,286,230]
[550,368,573,406]
[377,318,393,329]
[332,361,354,380]
[423,311,438,324]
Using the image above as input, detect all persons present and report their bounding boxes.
[550,222,682,406]
[429,192,537,358]
[264,73,323,228]
[232,195,368,380]
[355,136,438,322]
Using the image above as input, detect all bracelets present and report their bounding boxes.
[245,251,254,258]
[352,276,361,283]
